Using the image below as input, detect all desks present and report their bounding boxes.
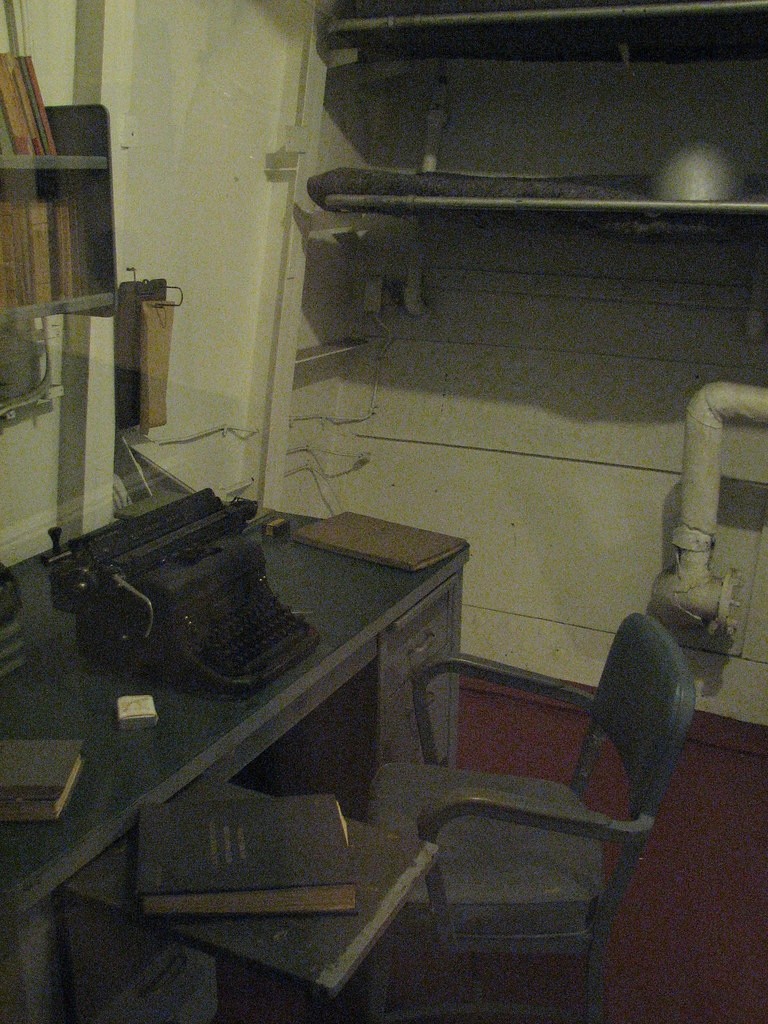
[1,503,465,1024]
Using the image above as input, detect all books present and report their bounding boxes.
[136,792,357,915]
[294,510,470,574]
[0,51,91,310]
[2,737,86,821]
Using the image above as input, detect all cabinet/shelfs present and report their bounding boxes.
[0,105,121,321]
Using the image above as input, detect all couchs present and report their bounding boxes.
[361,616,696,1024]
